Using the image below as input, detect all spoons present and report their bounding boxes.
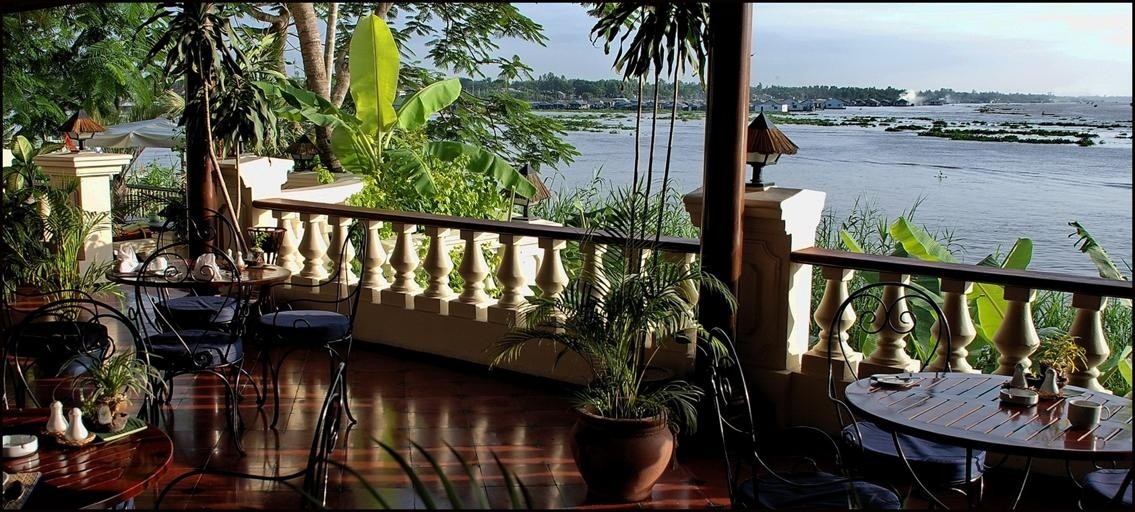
[2,478,24,511]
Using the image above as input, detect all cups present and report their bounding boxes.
[1067,399,1109,430]
[149,256,167,271]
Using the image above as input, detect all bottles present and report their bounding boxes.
[226,248,246,269]
[1011,363,1062,397]
[47,401,90,442]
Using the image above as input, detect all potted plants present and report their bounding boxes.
[73,351,165,432]
[1,201,117,386]
[480,247,739,503]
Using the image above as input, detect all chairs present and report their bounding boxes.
[132,240,262,436]
[254,221,369,430]
[824,283,991,510]
[157,206,241,258]
[707,327,900,512]
[6,301,151,421]
[154,358,354,510]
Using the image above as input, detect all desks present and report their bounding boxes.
[113,254,287,297]
[4,404,173,510]
[843,356,1135,510]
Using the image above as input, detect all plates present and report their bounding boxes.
[877,376,914,387]
